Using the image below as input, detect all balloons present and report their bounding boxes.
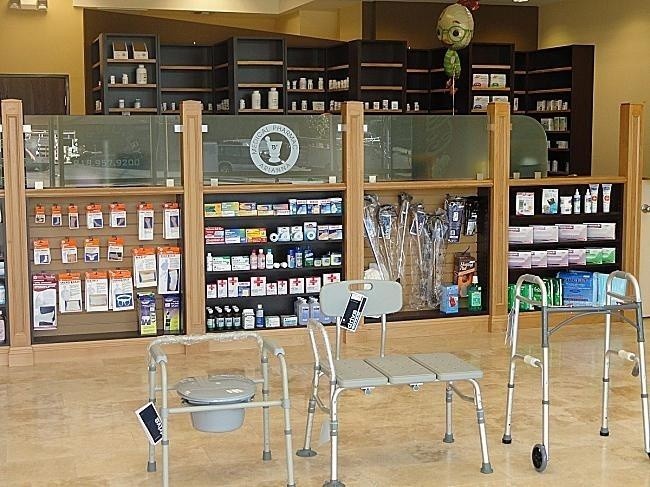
[434,0,480,94]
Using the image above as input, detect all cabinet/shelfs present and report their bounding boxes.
[514,50,528,115]
[495,179,636,329]
[430,41,514,114]
[528,44,592,176]
[215,35,287,115]
[25,190,199,341]
[90,32,159,116]
[0,190,12,347]
[356,185,508,324]
[190,187,357,335]
[287,44,328,114]
[159,42,215,113]
[407,47,430,116]
[327,39,410,115]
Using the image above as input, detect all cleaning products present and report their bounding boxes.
[573,189,581,214]
[584,189,592,214]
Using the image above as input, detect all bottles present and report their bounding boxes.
[573,187,591,215]
[135,63,147,84]
[300,77,307,90]
[122,73,128,86]
[204,295,333,333]
[133,98,141,109]
[330,100,341,111]
[406,101,419,111]
[239,99,246,109]
[117,99,124,109]
[267,87,279,110]
[171,101,176,111]
[249,246,304,271]
[307,79,313,90]
[329,79,346,89]
[251,90,261,109]
[205,250,212,273]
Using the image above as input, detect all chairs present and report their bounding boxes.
[300,275,497,487]
